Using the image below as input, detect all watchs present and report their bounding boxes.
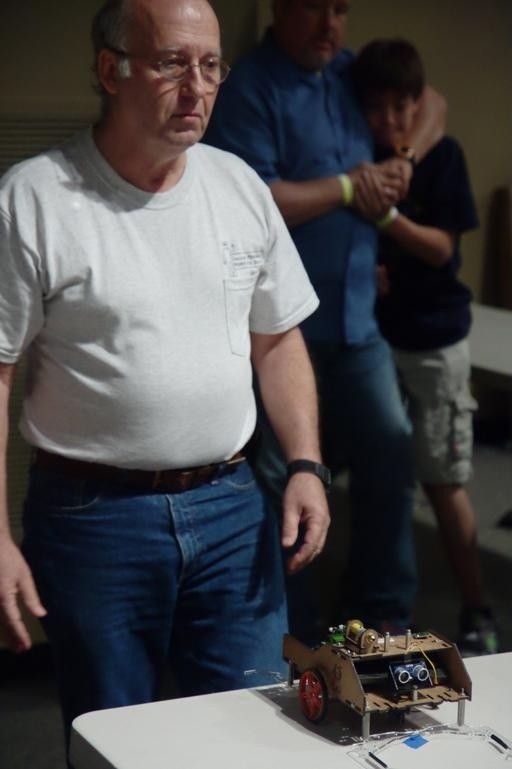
[288,458,334,491]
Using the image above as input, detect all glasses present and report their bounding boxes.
[113,48,231,85]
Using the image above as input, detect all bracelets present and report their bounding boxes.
[377,205,399,228]
[338,173,353,204]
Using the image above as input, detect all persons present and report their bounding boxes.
[347,34,493,650]
[203,1,421,637]
[0,0,332,761]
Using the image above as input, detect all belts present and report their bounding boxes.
[32,448,244,495]
